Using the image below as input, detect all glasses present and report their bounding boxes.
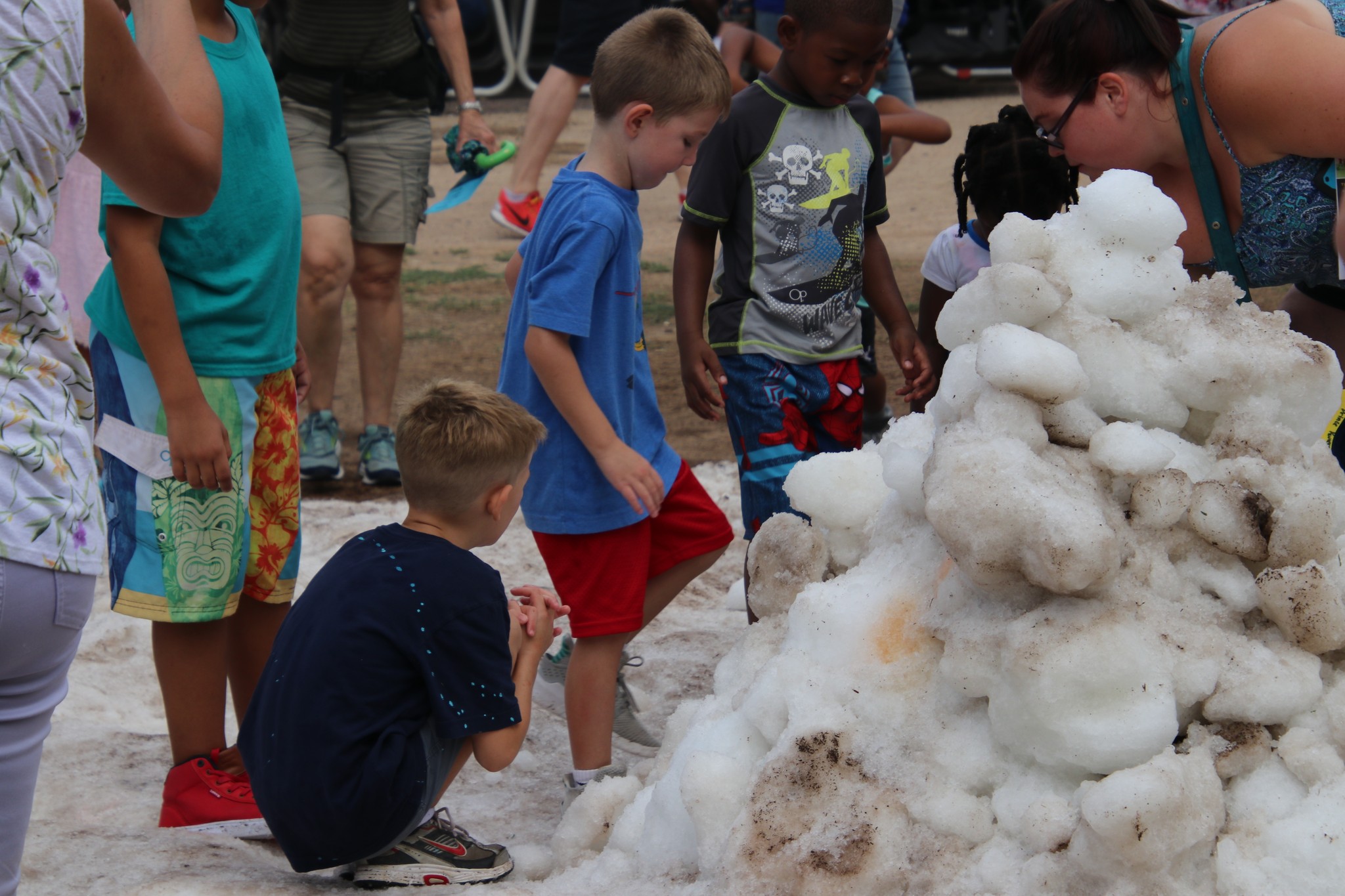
[1030,86,1092,151]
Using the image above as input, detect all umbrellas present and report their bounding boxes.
[442,123,515,176]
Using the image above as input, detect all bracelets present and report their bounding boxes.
[458,101,484,113]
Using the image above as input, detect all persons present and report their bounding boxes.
[84,0,312,840]
[230,0,495,487]
[671,0,939,540]
[0,0,221,896]
[1010,0,1345,471]
[236,377,573,885]
[496,7,734,802]
[909,103,1080,413]
[490,0,696,239]
[710,0,952,435]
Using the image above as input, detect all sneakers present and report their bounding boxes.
[537,632,664,749]
[157,746,278,843]
[298,405,345,482]
[490,183,542,238]
[337,804,516,886]
[677,192,690,219]
[352,424,405,488]
[556,759,629,809]
